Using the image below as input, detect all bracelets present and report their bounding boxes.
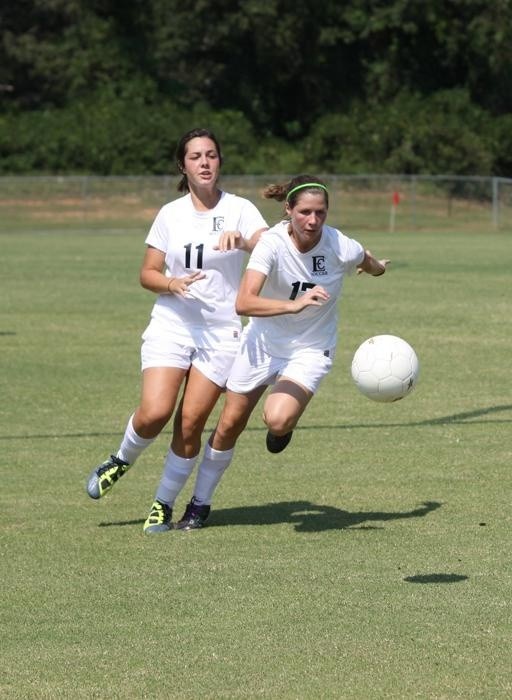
[372,267,386,277]
[165,277,177,294]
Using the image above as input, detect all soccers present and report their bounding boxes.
[352,335,419,404]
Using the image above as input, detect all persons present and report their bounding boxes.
[83,127,276,533]
[175,173,394,532]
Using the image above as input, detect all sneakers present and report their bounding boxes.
[176,496,210,531]
[87,454,133,499]
[267,430,292,453]
[143,498,172,536]
